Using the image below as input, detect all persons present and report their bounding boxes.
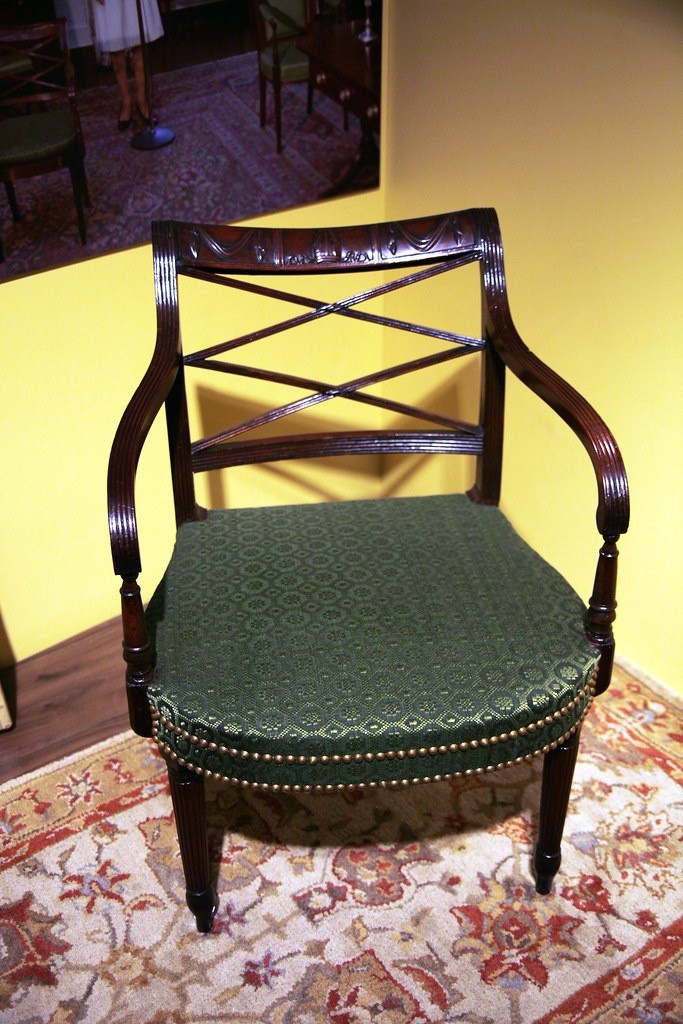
[87,0,166,134]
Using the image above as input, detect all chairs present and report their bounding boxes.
[254,0,350,155]
[105,206,633,937]
[0,17,90,264]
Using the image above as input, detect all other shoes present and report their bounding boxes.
[117,117,132,129]
[135,102,159,126]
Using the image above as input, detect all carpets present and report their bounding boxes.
[0,50,379,279]
[0,651,683,1024]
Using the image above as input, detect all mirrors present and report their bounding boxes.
[0,0,383,283]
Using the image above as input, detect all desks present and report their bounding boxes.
[297,46,381,140]
